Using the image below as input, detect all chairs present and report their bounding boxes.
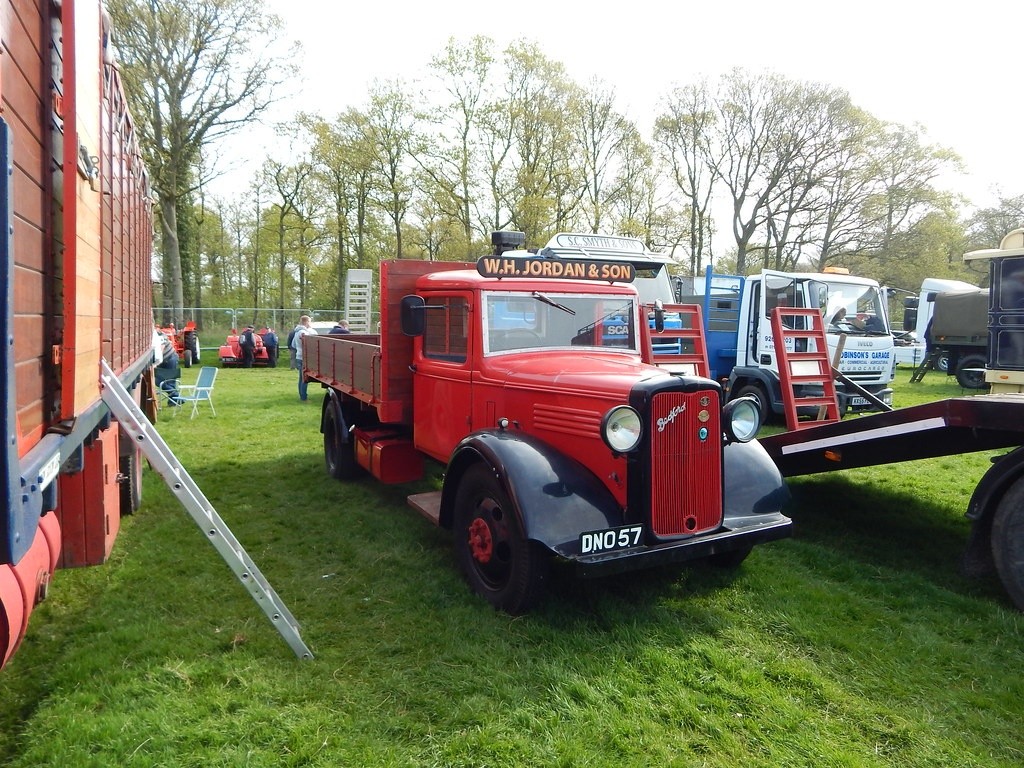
[219,336,269,368]
[172,366,218,419]
[156,379,181,414]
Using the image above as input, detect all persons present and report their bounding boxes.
[262,325,278,369]
[286,321,300,370]
[152,328,184,407]
[238,325,256,368]
[291,314,318,401]
[327,320,352,334]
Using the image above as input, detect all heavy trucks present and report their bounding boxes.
[0,0,153,662]
[880,278,983,371]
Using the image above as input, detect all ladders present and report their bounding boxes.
[100,358,314,660]
[909,347,943,383]
[344,269,373,335]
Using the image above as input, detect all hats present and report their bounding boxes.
[339,320,349,326]
[248,326,253,328]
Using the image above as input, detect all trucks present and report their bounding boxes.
[485,232,684,356]
[675,263,919,421]
[963,228,1024,394]
[932,290,991,390]
[300,252,791,604]
[754,306,1023,607]
